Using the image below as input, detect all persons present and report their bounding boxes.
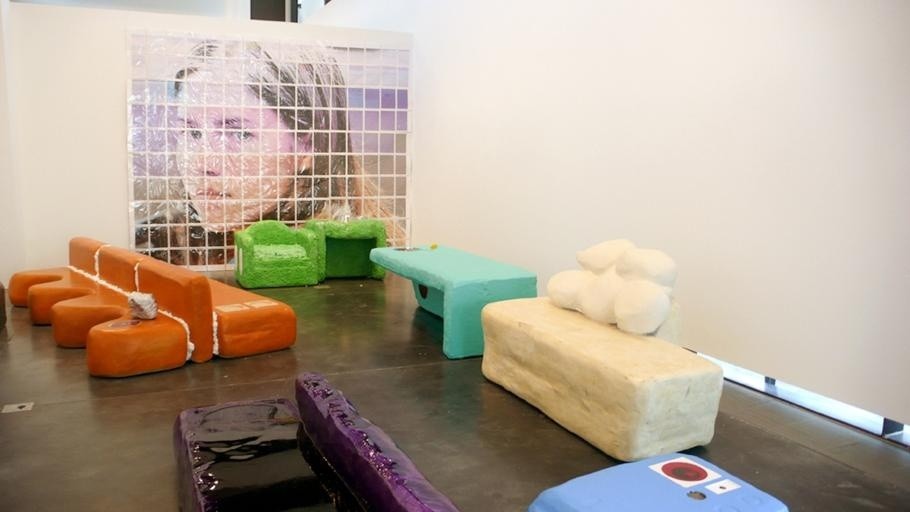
[132,38,407,268]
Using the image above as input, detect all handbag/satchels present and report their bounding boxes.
[127,291,158,319]
